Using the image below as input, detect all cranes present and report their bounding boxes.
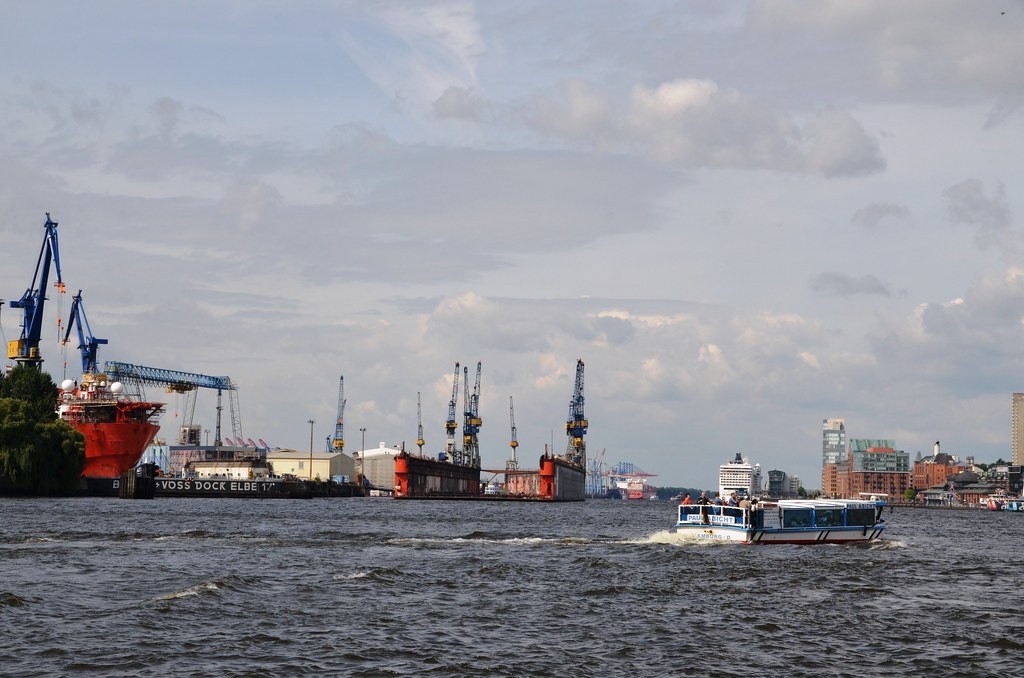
[8,214,68,369]
[60,289,107,397]
[464,361,482,467]
[416,392,426,457]
[565,358,590,467]
[330,374,348,452]
[509,395,519,467]
[445,362,461,462]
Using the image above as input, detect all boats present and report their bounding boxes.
[649,496,659,501]
[676,493,889,544]
[626,481,657,500]
[58,369,168,499]
[719,450,765,501]
[671,495,683,502]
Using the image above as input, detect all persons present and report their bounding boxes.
[697,492,710,504]
[681,493,693,505]
[722,494,758,529]
[714,492,722,505]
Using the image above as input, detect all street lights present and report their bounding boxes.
[205,429,210,446]
[306,419,317,481]
[360,426,367,487]
